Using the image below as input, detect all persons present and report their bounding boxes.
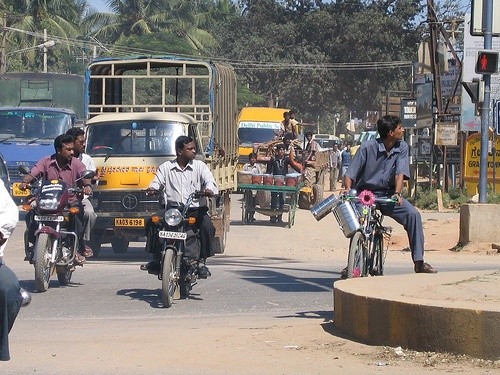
[39,127,100,257]
[277,112,317,149]
[341,144,352,188]
[301,131,321,169]
[140,136,218,279]
[240,153,262,223]
[266,144,288,224]
[341,140,348,153]
[0,178,24,361]
[273,110,301,142]
[281,138,296,173]
[329,143,343,191]
[339,115,438,279]
[19,134,93,266]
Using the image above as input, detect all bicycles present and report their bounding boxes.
[341,188,402,279]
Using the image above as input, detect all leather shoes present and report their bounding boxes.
[197,265,211,277]
[148,258,160,275]
[416,263,437,273]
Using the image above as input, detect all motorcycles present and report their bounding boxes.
[17,164,93,293]
[140,187,213,308]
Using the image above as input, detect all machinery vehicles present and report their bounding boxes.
[251,137,332,211]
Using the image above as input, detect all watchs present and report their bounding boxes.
[394,191,403,197]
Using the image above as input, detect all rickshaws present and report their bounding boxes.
[236,171,304,230]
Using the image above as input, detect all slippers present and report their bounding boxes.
[81,245,93,258]
[73,254,86,266]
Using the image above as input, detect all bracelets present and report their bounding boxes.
[83,183,93,189]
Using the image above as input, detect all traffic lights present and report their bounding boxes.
[474,49,499,74]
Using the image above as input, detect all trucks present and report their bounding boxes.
[237,107,304,170]
[82,55,238,258]
[0,72,85,206]
